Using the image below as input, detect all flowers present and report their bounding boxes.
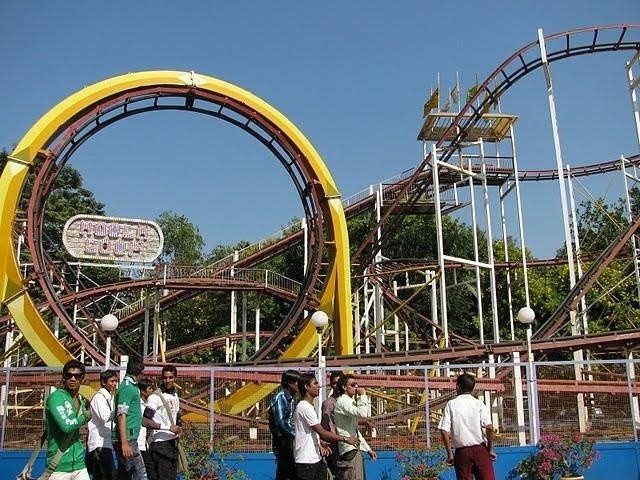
[500,431,603,480]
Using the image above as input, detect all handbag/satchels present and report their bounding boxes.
[15,465,49,480]
[176,442,187,472]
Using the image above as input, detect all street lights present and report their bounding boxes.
[99,314,119,370]
[517,305,538,446]
[310,308,329,448]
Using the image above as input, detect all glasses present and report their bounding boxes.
[64,372,82,381]
[346,382,357,387]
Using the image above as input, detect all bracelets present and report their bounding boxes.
[160,422,171,431]
[343,435,346,443]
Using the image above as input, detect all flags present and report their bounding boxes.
[424,82,500,123]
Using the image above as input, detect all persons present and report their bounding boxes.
[293,374,360,479]
[141,365,183,480]
[137,379,157,480]
[321,371,346,480]
[113,354,148,480]
[334,375,378,480]
[43,359,92,480]
[268,369,301,480]
[437,374,497,480]
[86,368,118,480]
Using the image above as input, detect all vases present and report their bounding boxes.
[559,475,585,480]
